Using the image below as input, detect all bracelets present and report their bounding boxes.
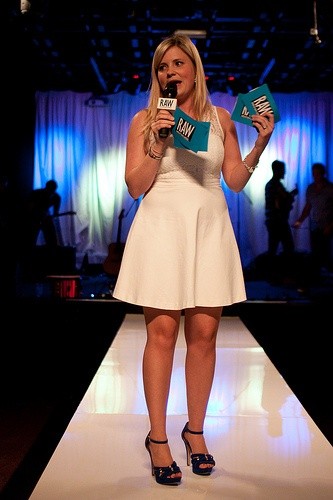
[148,145,164,160]
[243,157,258,173]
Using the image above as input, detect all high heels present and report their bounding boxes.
[181,422,215,476]
[145,431,182,485]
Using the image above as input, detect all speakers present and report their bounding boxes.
[25,246,76,275]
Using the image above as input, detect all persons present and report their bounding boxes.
[109,34,275,484]
[29,178,62,248]
[266,158,333,285]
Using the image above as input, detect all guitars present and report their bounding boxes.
[102,208,125,275]
[44,210,76,220]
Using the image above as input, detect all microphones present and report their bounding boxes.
[159,81,178,139]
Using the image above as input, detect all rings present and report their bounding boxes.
[262,124,268,129]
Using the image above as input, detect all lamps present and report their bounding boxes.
[124,73,142,96]
[228,75,249,97]
